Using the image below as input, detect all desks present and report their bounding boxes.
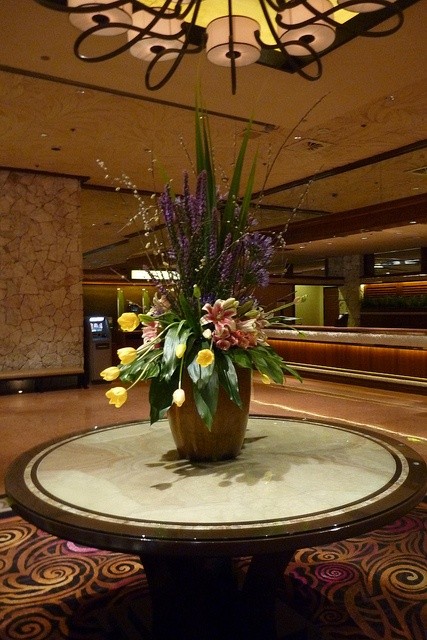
[0,415,427,638]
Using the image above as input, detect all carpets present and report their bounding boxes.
[0,491,427,640]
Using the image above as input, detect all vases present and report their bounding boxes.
[166,364,251,458]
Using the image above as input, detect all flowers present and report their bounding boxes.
[90,46,329,426]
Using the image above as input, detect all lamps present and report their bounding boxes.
[41,0,422,95]
